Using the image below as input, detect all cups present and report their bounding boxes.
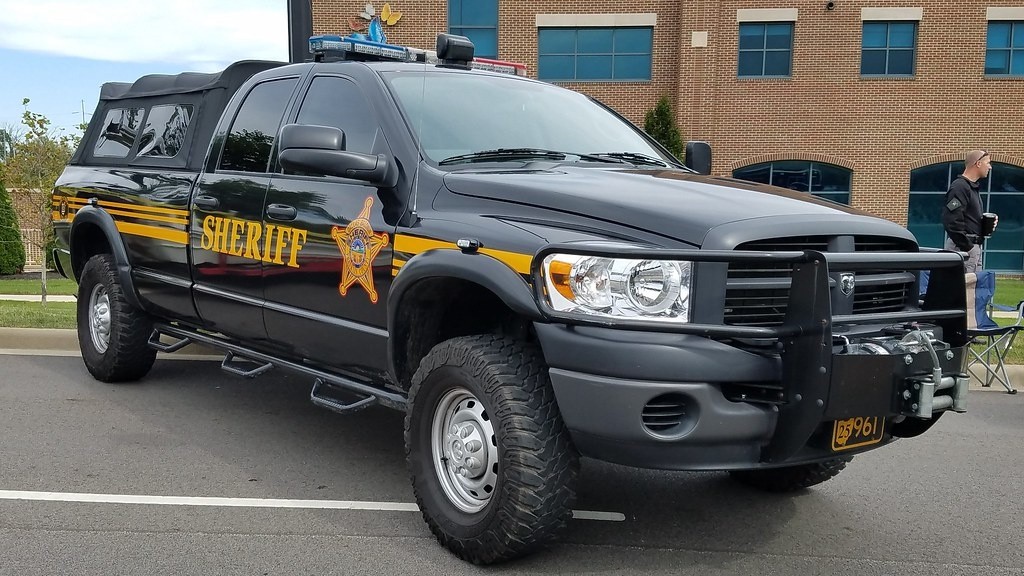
[981,213,996,235]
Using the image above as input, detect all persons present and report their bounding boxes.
[940,151,999,344]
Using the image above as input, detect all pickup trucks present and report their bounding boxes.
[46,30,979,566]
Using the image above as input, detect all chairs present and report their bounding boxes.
[919,270,1024,394]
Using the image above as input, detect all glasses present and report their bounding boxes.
[973,151,990,166]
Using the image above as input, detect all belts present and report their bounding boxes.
[968,236,984,244]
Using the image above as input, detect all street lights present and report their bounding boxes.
[72,100,92,126]
